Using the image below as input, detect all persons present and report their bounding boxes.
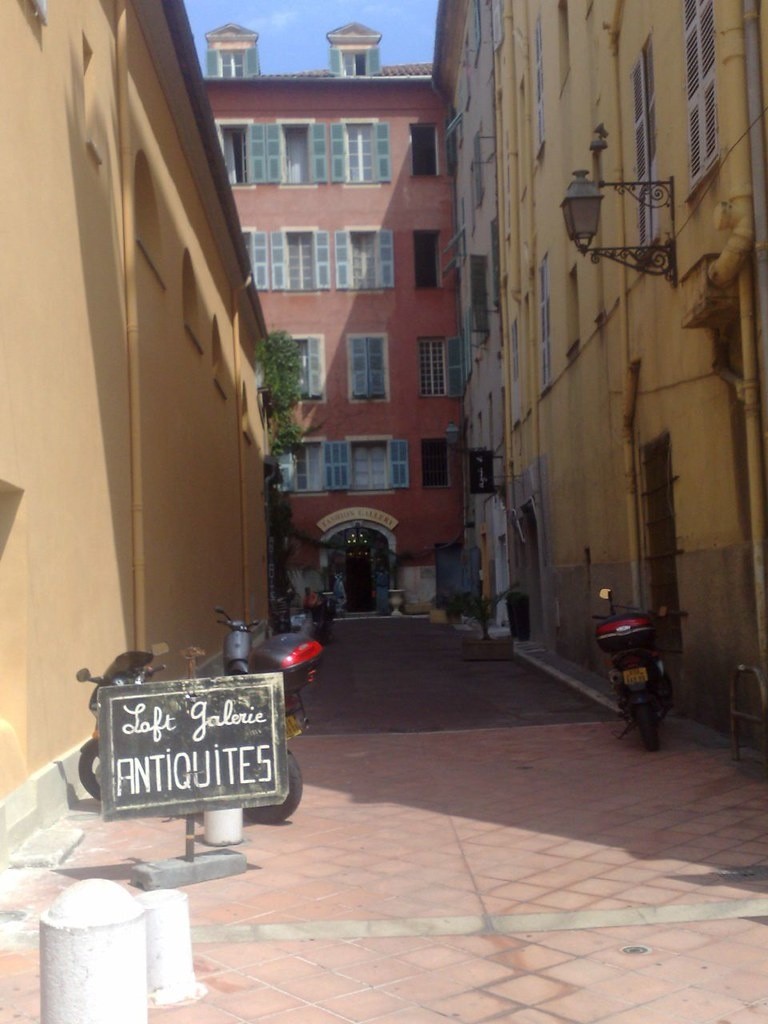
[334,570,346,599]
[303,587,318,625]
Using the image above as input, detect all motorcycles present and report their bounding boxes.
[213,607,259,676]
[76,634,323,825]
[311,591,344,644]
[594,587,690,750]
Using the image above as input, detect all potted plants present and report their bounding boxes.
[461,582,520,661]
[387,563,406,615]
[429,592,460,624]
[506,592,529,641]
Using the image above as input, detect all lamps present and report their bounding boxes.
[559,170,678,291]
[443,418,487,451]
